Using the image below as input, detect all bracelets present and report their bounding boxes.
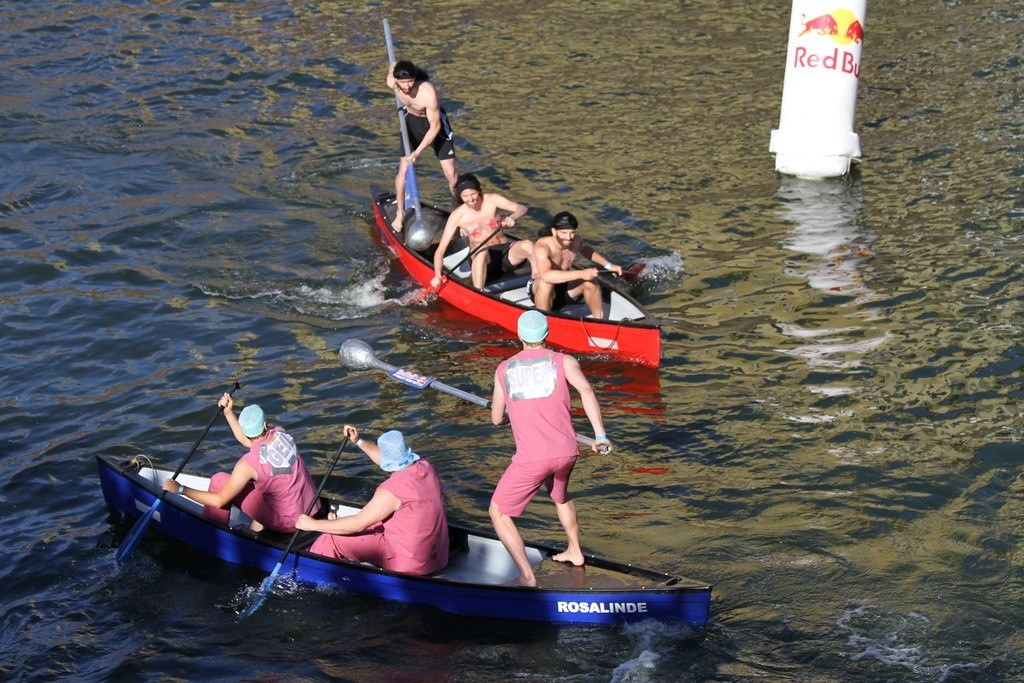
[595,434,607,440]
[604,262,613,270]
[355,437,364,447]
[177,484,186,496]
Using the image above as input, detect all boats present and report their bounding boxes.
[371,182,661,371]
[95,452,714,633]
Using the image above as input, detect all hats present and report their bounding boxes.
[377,430,420,472]
[238,404,265,438]
[518,310,548,343]
[453,173,480,197]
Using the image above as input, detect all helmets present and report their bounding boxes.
[393,60,417,79]
[552,211,578,230]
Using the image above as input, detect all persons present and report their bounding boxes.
[487,309,614,587]
[429,172,538,290]
[294,423,449,576]
[161,391,322,533]
[386,59,460,233]
[526,210,624,320]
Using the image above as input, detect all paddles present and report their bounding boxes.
[414,219,506,303]
[116,379,241,566]
[597,262,647,284]
[235,428,354,620]
